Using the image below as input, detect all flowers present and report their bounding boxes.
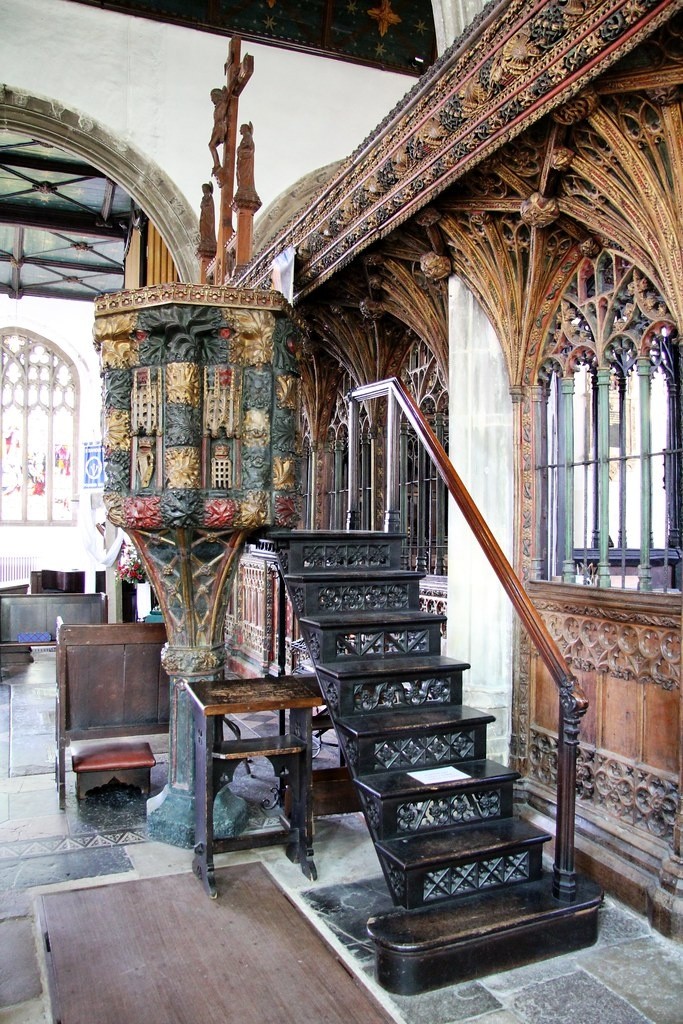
[114,537,161,611]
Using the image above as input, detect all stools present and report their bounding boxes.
[70,740,156,802]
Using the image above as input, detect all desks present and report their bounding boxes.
[184,674,362,899]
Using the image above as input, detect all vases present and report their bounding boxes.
[145,611,164,623]
